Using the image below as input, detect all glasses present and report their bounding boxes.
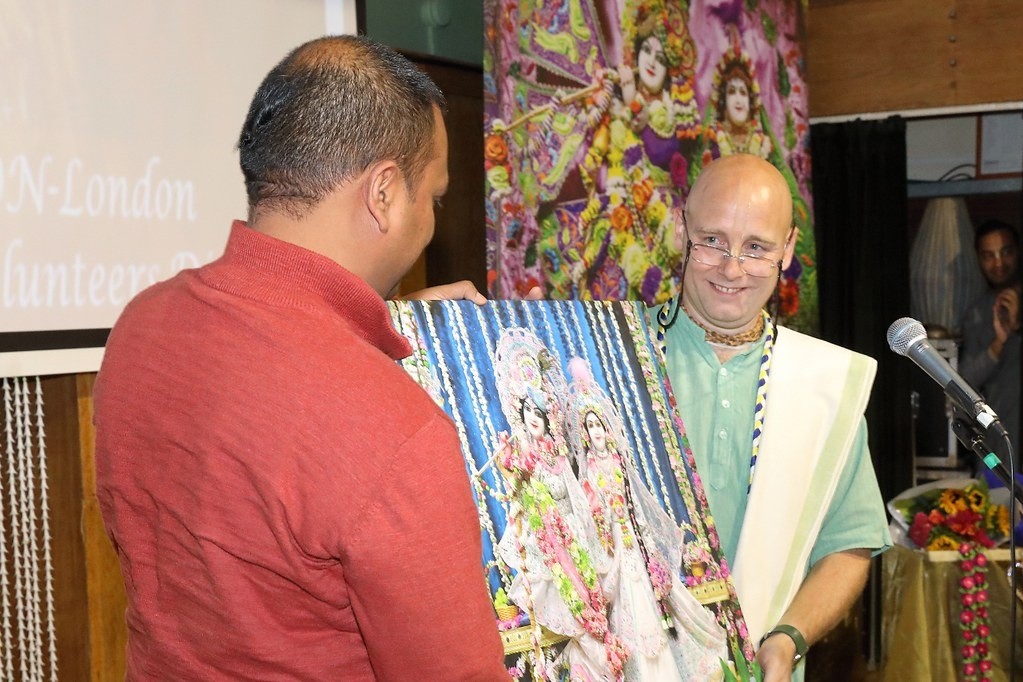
[682,209,796,277]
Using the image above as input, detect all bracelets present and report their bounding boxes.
[760,625,811,668]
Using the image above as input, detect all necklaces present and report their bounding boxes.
[680,301,766,345]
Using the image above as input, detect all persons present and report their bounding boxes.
[646,154,879,682]
[956,218,1023,474]
[96,36,515,682]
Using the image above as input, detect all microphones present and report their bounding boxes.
[887,317,1009,437]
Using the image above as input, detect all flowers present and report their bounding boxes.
[906,482,1009,553]
